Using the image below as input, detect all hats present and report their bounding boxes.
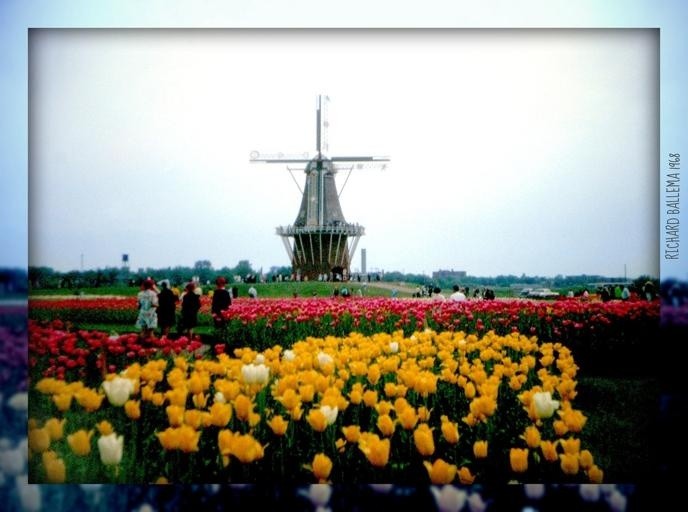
[216,278,225,286]
[140,280,155,291]
[184,284,195,292]
